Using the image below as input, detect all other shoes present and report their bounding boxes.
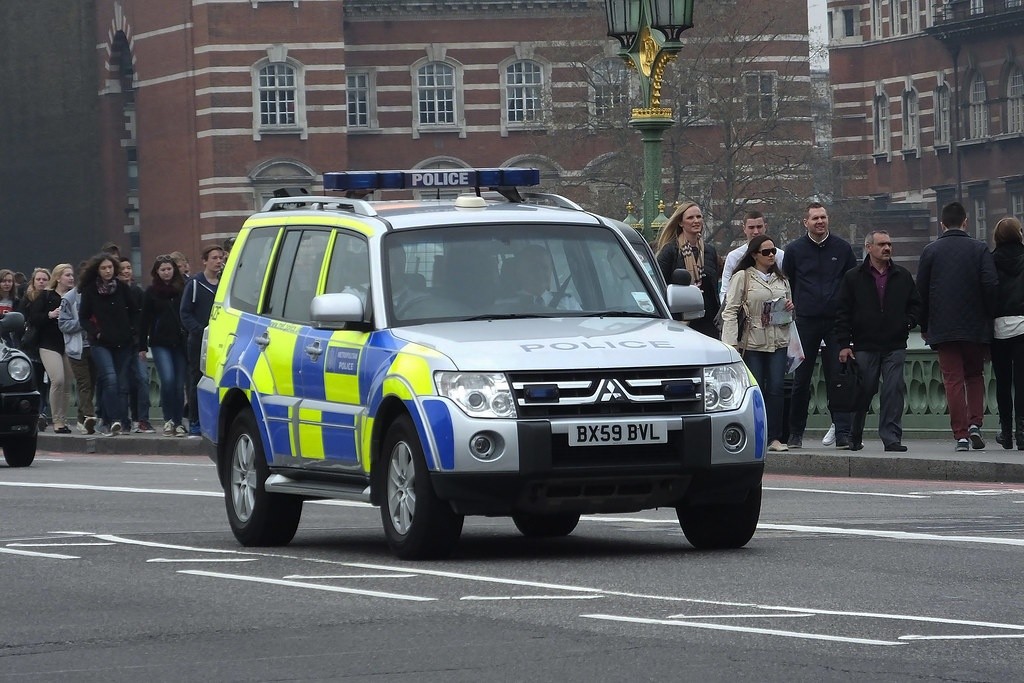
[885,443,907,452]
[76,417,96,435]
[123,427,131,435]
[835,437,849,450]
[995,430,1013,449]
[54,425,71,433]
[849,443,864,452]
[38,416,47,432]
[97,421,122,436]
[787,435,803,447]
[1017,444,1024,449]
[769,439,788,451]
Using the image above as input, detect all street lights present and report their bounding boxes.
[604,0,695,242]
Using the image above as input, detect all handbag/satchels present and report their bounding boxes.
[713,267,749,342]
[21,319,40,347]
[827,353,867,414]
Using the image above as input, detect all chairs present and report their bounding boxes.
[501,258,521,296]
[432,255,462,287]
[388,245,426,292]
[318,251,365,294]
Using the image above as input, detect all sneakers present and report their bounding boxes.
[969,425,985,449]
[174,425,187,436]
[821,423,837,446]
[131,420,157,433]
[162,420,175,436]
[956,438,969,451]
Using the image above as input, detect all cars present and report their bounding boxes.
[0,311,41,467]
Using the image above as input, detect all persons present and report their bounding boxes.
[340,238,447,322]
[985,217,1024,451]
[781,201,860,448]
[656,201,795,453]
[914,201,1007,453]
[836,229,919,452]
[493,245,584,312]
[0,238,235,439]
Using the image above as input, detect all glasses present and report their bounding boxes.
[156,254,171,262]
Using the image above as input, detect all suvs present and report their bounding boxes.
[198,168,768,560]
